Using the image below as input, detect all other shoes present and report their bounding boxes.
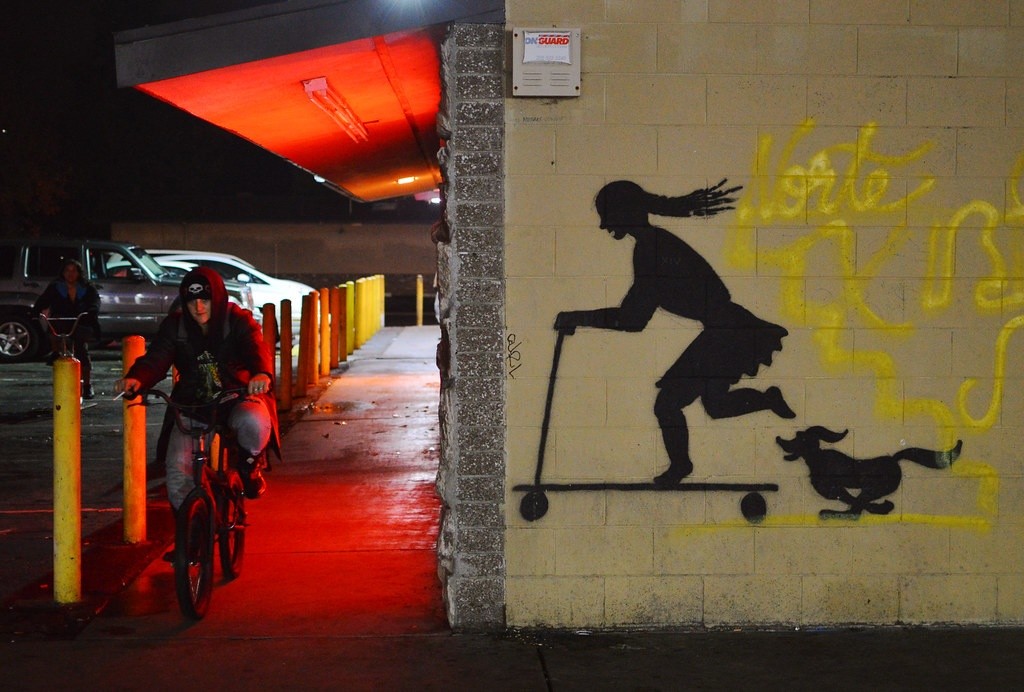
[163,525,201,565]
[238,447,266,499]
[45,351,60,365]
[83,385,95,399]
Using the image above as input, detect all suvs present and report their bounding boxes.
[0,229,244,364]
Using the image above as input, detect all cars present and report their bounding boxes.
[94,248,333,353]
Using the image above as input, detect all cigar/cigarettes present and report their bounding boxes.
[114,391,125,400]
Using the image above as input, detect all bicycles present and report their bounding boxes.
[40,312,89,359]
[122,387,247,620]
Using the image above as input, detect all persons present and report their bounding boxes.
[29,258,101,399]
[114,266,281,561]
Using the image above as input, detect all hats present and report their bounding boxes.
[180,275,210,303]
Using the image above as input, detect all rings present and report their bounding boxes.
[254,389,257,391]
[258,387,262,390]
